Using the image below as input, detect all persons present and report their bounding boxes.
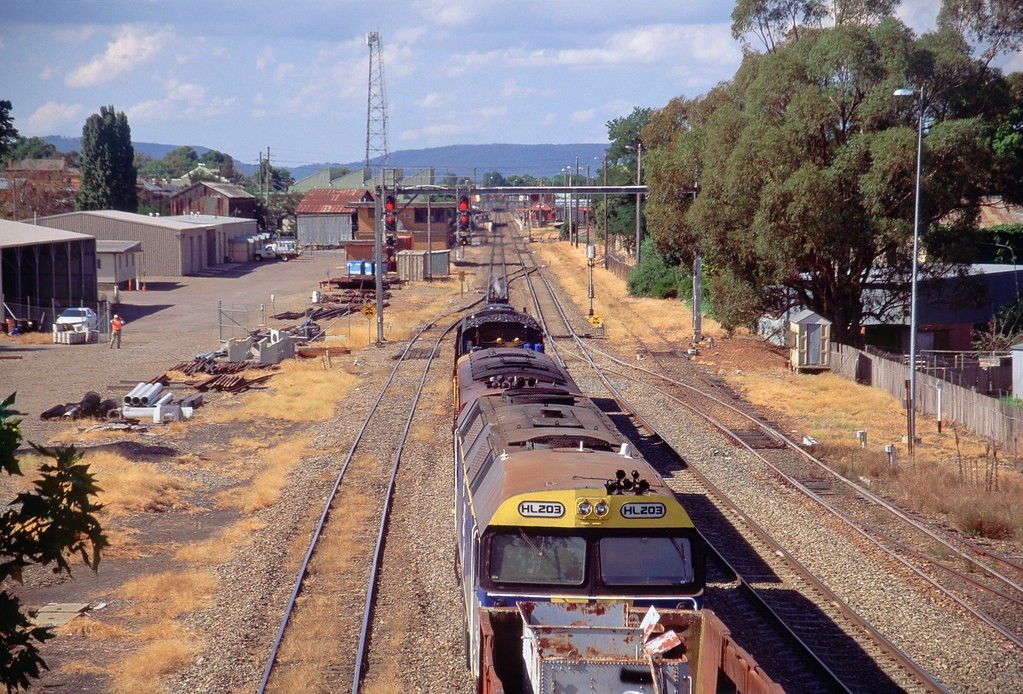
[109,314,124,349]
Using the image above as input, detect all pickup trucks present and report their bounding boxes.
[253,240,298,262]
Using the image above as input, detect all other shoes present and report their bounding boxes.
[108,346,111,348]
[117,346,120,349]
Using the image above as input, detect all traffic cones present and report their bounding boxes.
[127,277,133,291]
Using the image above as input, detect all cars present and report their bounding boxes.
[56,307,98,331]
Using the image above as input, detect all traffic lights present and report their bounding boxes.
[386,236,394,246]
[461,238,468,245]
[384,192,395,212]
[385,214,396,231]
[458,193,470,213]
[460,215,469,230]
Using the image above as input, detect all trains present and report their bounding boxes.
[450,289,787,694]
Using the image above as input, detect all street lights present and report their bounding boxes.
[566,165,572,247]
[624,142,641,267]
[680,182,700,344]
[574,156,584,249]
[594,153,608,269]
[561,168,567,240]
[891,86,923,444]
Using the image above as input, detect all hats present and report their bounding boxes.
[113,315,118,319]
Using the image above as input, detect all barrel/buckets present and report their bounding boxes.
[52,324,99,344]
[312,291,321,303]
[6,319,17,333]
[17,320,28,327]
